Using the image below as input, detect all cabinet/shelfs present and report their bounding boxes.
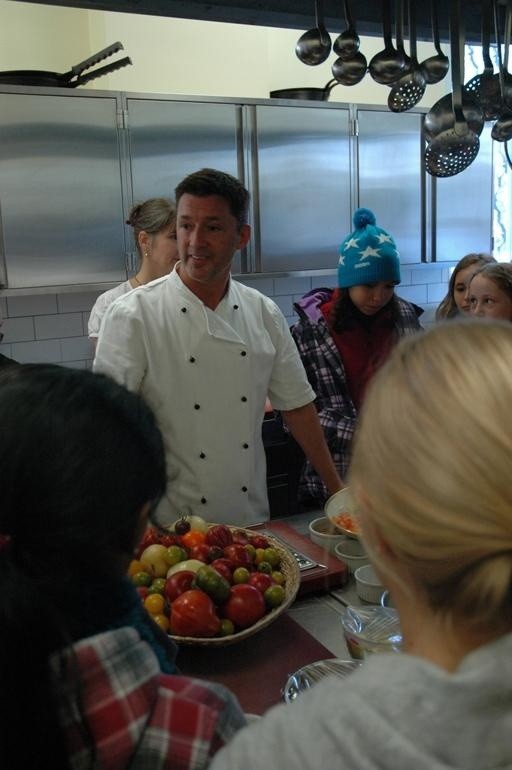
[355,104,494,271]
[120,92,355,280]
[0,84,131,298]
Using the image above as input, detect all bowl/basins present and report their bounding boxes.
[282,482,401,705]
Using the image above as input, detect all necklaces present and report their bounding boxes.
[132,276,141,286]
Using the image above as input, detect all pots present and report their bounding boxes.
[0,40,134,105]
[268,78,346,100]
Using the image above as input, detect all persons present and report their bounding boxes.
[89,168,347,530]
[201,314,512,770]
[438,255,498,326]
[2,362,243,767]
[467,262,512,320]
[274,208,427,516]
[89,196,182,354]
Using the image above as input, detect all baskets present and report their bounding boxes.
[160,521,302,650]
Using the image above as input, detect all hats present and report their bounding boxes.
[337,208,401,289]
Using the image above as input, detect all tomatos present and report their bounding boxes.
[128,516,286,636]
